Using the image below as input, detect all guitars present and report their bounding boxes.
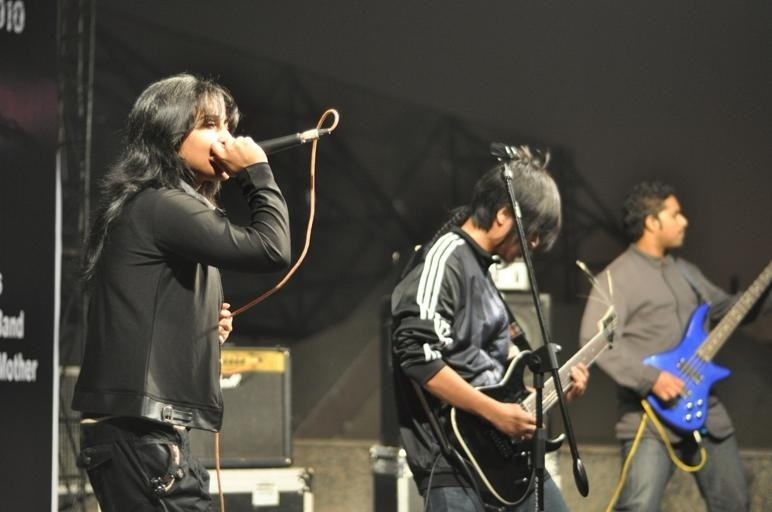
[639,263,772,433]
[445,304,618,506]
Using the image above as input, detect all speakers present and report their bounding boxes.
[377,290,552,447]
[189,342,294,470]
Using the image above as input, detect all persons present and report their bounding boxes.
[389,146,590,511]
[580,178,771,512]
[70,72,292,511]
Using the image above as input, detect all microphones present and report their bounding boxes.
[256,128,330,156]
